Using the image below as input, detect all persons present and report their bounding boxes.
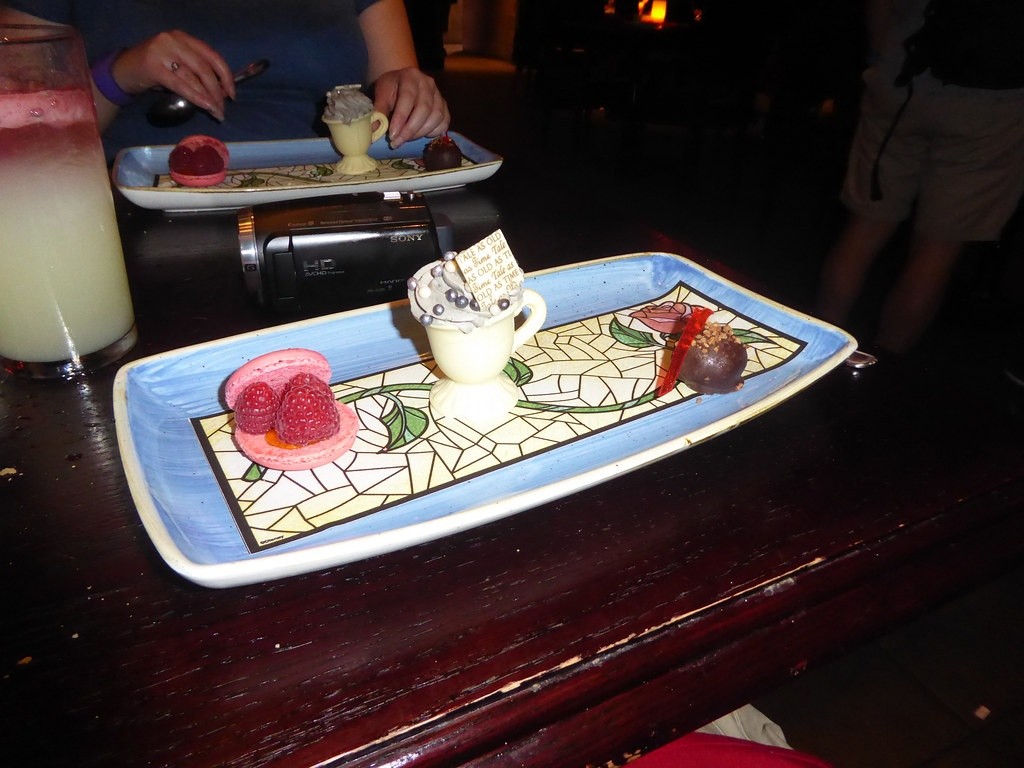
[8,1,451,167]
[818,0,1024,358]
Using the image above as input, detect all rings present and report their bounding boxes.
[171,61,182,74]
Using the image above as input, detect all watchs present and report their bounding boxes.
[91,45,138,105]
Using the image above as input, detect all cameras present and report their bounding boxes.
[237,191,454,316]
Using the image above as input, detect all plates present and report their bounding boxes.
[111,253,858,587]
[113,132,503,212]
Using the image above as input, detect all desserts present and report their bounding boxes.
[168,133,230,187]
[658,308,748,397]
[423,133,463,172]
[224,348,359,471]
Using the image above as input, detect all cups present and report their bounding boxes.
[0,23,137,377]
[323,107,388,174]
[408,261,546,420]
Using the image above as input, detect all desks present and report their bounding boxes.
[1,190,1024,768]
